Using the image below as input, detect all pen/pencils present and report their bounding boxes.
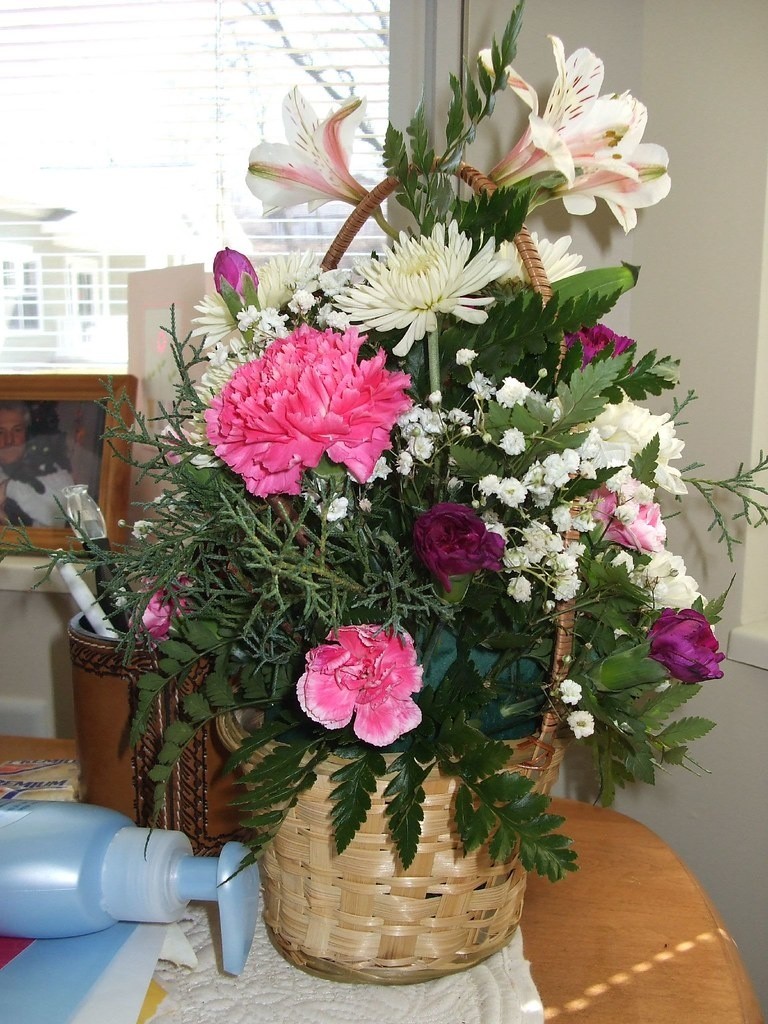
[46,482,133,645]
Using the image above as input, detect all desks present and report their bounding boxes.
[0,734,760,1024]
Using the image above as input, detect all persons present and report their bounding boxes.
[0,401,74,529]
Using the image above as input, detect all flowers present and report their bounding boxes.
[0,0,768,880]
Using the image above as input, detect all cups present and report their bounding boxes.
[66,613,255,856]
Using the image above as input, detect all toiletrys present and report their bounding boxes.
[0,798,261,977]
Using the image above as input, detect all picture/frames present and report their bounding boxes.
[3,372,138,551]
